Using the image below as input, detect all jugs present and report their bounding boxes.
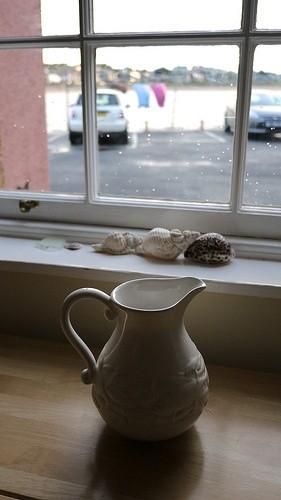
[60,274,212,442]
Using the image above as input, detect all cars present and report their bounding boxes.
[225,94,280,137]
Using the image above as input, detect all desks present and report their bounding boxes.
[1,334,280,499]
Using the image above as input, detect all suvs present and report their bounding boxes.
[71,90,129,146]
